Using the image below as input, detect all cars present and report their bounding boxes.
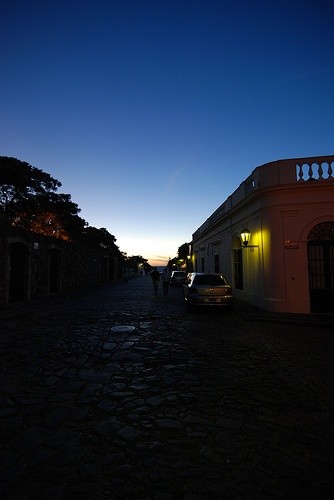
[170,271,187,287]
[183,272,234,313]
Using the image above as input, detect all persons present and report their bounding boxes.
[151,267,161,296]
[122,268,129,289]
[161,268,171,295]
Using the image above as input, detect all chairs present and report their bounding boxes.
[199,277,208,285]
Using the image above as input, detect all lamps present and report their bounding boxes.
[187,255,191,260]
[241,229,258,247]
